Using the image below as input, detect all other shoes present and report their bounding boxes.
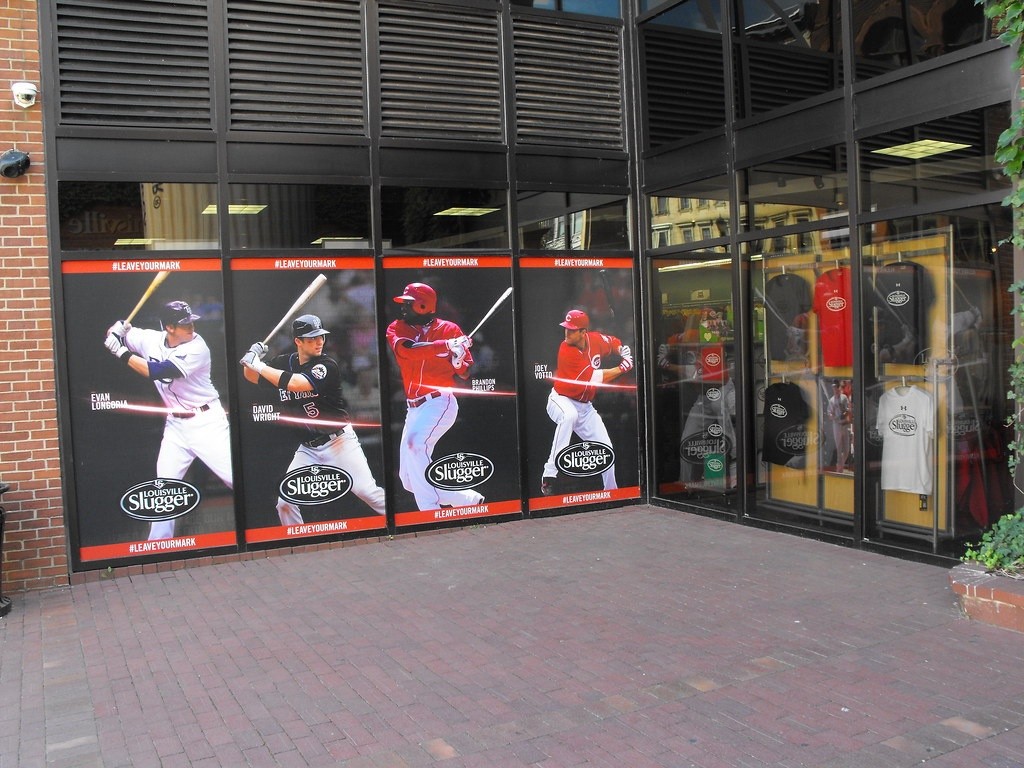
[837,466,844,473]
[472,493,486,505]
[729,463,737,488]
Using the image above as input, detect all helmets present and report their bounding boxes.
[394,282,437,315]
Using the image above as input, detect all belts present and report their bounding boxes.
[409,391,441,407]
[172,403,210,418]
[305,429,344,447]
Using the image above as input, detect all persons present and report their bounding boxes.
[827,379,850,473]
[104,301,233,541]
[243,314,385,525]
[541,309,633,496]
[385,283,486,510]
[658,329,735,486]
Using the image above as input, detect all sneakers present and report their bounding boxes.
[541,476,558,496]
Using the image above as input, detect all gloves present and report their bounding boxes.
[619,345,631,357]
[444,334,473,350]
[970,306,982,329]
[239,352,267,374]
[658,344,670,358]
[109,320,132,342]
[655,355,670,370]
[249,341,269,359]
[618,355,634,374]
[450,344,466,369]
[104,332,129,359]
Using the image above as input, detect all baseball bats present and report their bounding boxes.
[468,286,512,339]
[820,379,835,411]
[599,269,628,347]
[264,273,328,346]
[126,271,170,322]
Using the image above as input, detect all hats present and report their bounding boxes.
[702,308,718,320]
[293,314,330,338]
[160,300,201,331]
[831,380,840,387]
[559,310,589,330]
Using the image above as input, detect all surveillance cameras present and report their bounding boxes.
[11,82,37,104]
[0,151,29,181]
